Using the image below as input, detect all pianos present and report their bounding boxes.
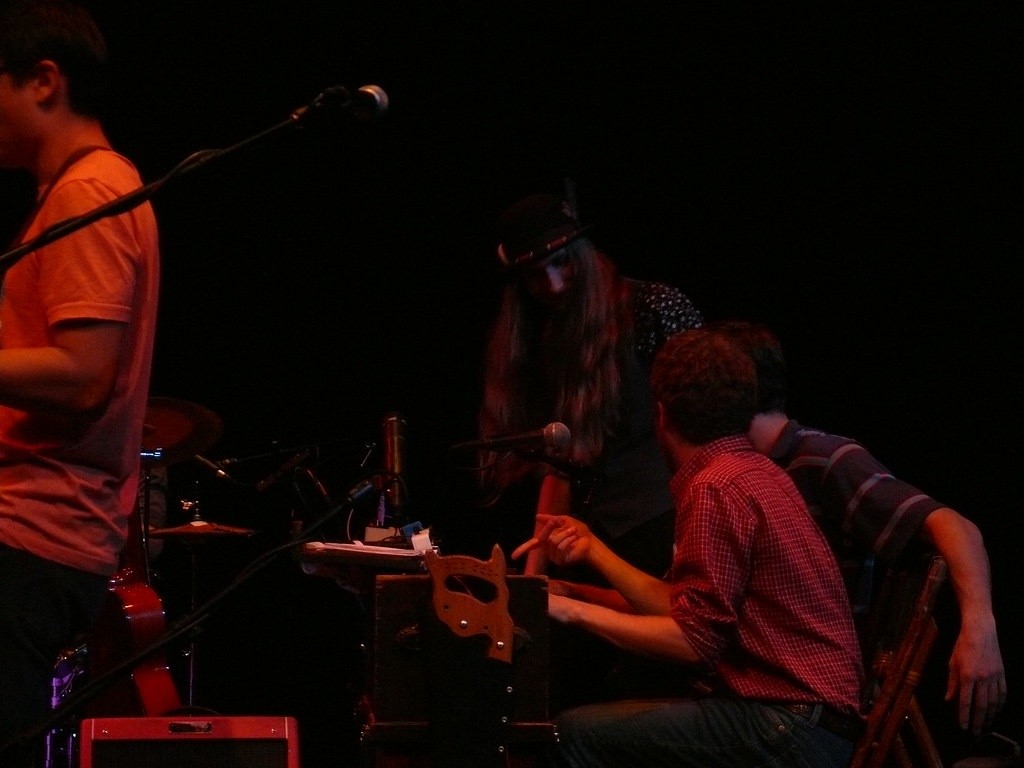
[294,540,563,768]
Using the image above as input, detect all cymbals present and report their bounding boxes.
[148,518,255,541]
[142,396,224,468]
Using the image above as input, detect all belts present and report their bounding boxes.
[772,692,866,744]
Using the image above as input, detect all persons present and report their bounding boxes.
[0,0,163,768]
[512,333,865,768]
[482,195,707,768]
[721,328,1008,736]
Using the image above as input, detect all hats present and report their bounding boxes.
[499,193,589,268]
[145,394,225,462]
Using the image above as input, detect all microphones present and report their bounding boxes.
[383,411,402,517]
[257,450,312,492]
[192,454,233,480]
[344,86,389,119]
[483,423,571,456]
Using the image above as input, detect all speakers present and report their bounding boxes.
[82,716,302,768]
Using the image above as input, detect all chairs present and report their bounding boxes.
[822,547,947,768]
